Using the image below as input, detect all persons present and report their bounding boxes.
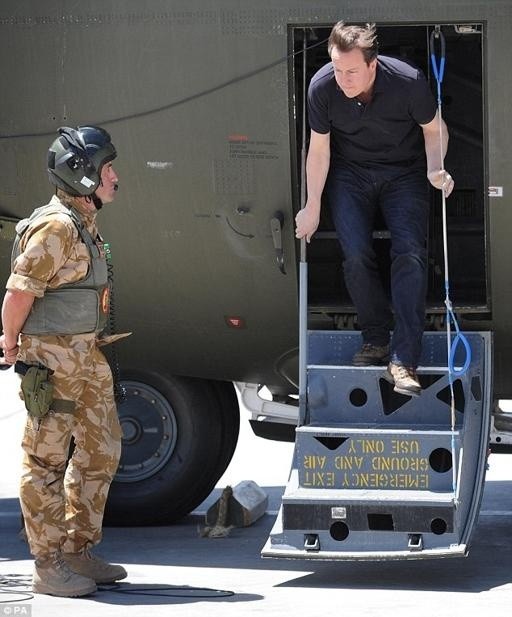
[292,24,455,398]
[0,125,128,599]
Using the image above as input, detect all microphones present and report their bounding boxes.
[114,184,118,191]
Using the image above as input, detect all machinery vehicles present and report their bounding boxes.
[0,0,510,564]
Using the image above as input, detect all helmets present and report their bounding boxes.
[45,124,118,198]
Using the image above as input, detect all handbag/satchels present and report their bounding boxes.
[20,365,54,419]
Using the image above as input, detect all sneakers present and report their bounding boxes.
[31,560,98,596]
[382,361,422,396]
[352,343,391,367]
[66,556,127,585]
